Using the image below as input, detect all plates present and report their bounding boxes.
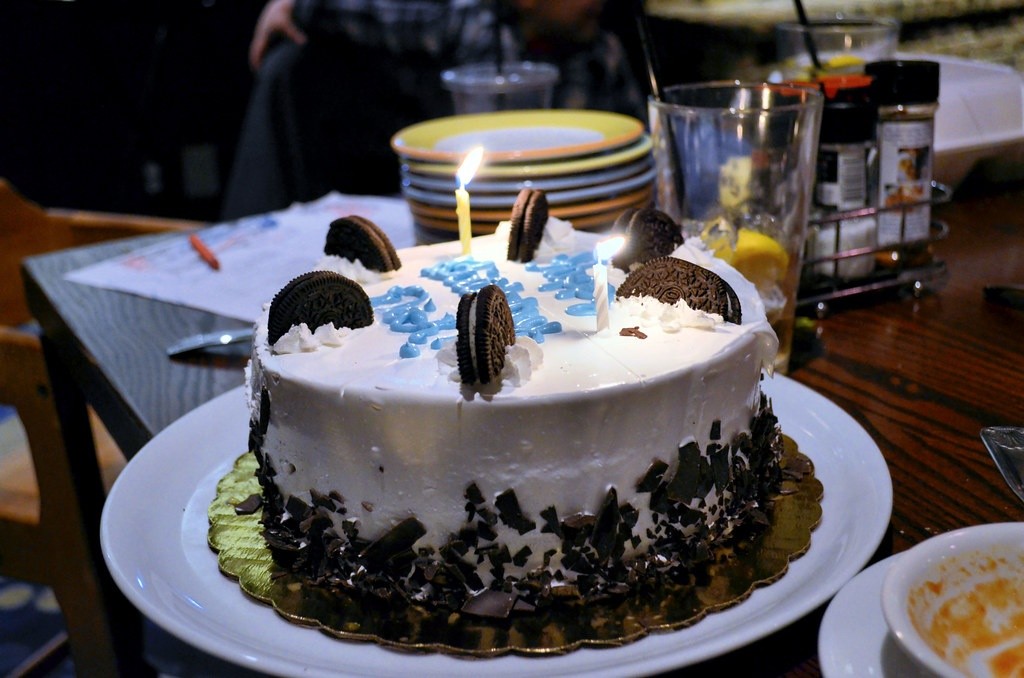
[389,109,659,237]
[99,383,892,677]
[817,550,935,677]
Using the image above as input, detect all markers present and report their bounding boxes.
[188,233,220,270]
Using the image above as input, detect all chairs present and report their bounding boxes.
[1,180,208,678]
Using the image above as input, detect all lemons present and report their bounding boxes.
[712,232,787,284]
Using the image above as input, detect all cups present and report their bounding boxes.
[772,12,900,76]
[441,62,559,115]
[646,80,825,373]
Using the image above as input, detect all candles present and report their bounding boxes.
[454,145,486,254]
[592,236,627,333]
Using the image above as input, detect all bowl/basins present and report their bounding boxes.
[885,522,1024,677]
[787,50,1024,187]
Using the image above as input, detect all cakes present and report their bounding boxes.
[243,188,782,624]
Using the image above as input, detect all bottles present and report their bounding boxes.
[803,58,941,277]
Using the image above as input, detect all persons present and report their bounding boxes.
[248,1,647,223]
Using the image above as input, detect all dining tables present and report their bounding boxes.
[26,190,1024,678]
